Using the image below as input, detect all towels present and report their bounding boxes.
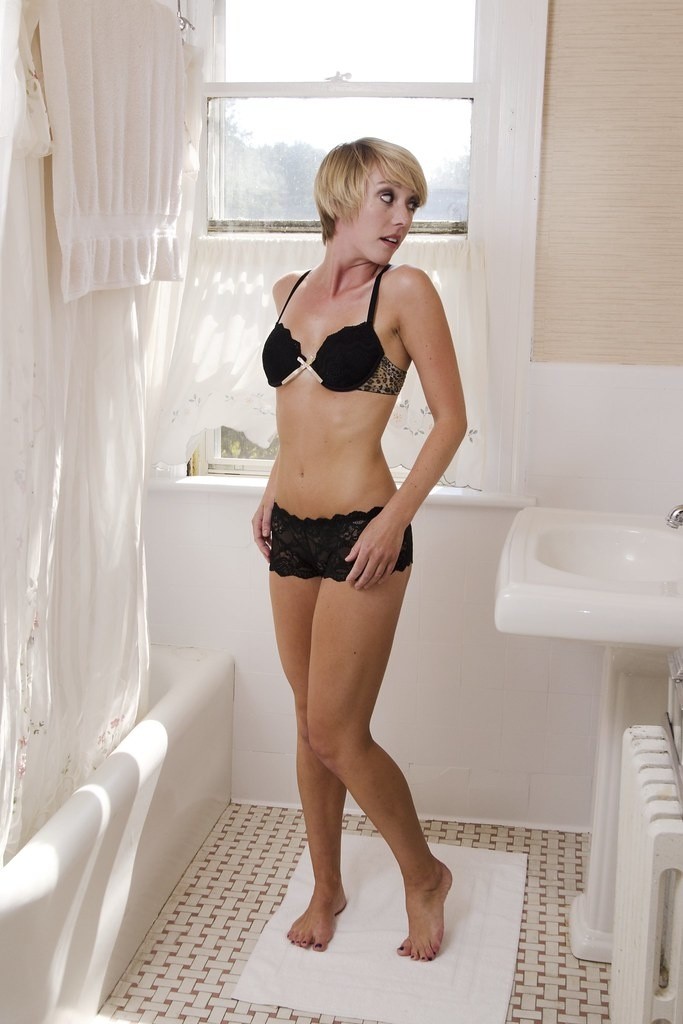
[38,1,184,301]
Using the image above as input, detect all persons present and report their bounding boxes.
[252,135,469,961]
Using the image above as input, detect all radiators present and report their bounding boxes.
[606,721,683,1023]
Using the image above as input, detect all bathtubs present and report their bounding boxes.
[491,504,683,647]
[1,643,234,1021]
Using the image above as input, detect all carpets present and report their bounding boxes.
[230,829,529,1023]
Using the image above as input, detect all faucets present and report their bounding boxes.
[667,505,682,530]
